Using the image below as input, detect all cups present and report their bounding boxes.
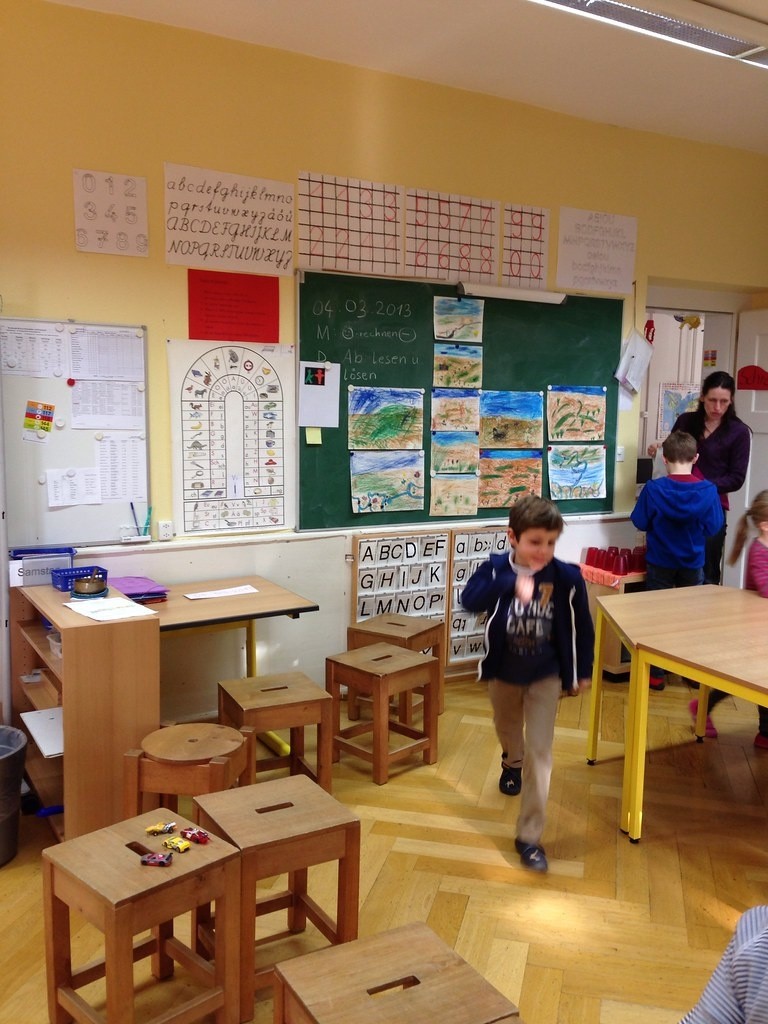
[585,546,645,576]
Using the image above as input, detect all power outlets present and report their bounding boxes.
[157,520,173,541]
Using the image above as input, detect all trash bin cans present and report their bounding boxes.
[0,724,28,868]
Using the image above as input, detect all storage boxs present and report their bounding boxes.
[8,547,78,568]
[40,668,62,705]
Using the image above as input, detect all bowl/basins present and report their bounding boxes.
[46,634,62,654]
[74,579,105,593]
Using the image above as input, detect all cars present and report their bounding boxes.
[162,836,192,853]
[180,827,211,845]
[140,852,174,868]
[145,821,177,836]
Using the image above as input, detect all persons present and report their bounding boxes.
[648,371,750,584]
[461,495,595,874]
[690,489,768,747]
[676,905,768,1024]
[629,430,724,691]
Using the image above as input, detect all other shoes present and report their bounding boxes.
[649,675,665,690]
[688,700,718,738]
[515,837,548,873]
[499,768,521,795]
[754,732,768,749]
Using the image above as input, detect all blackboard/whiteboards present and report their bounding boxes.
[293,268,626,532]
[1,317,153,551]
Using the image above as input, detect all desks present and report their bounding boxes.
[139,575,320,757]
[585,584,768,843]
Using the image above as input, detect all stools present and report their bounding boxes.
[41,808,241,1024]
[325,641,440,785]
[218,671,333,795]
[123,718,256,827]
[274,920,519,1024]
[193,773,362,1024]
[347,613,445,726]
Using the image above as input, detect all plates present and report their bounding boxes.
[70,586,108,598]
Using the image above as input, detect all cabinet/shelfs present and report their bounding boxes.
[578,562,647,682]
[9,584,160,844]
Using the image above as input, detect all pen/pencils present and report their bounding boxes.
[142,506,152,536]
[130,502,141,536]
